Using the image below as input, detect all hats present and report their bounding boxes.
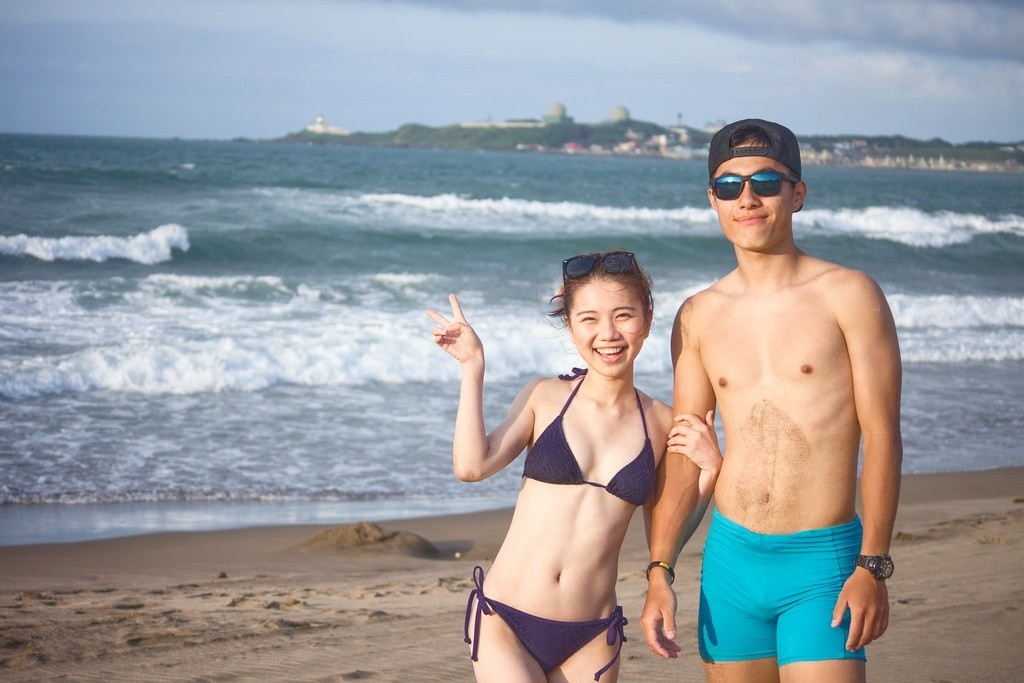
[709,119,801,179]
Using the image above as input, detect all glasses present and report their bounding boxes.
[562,252,645,289]
[713,172,800,201]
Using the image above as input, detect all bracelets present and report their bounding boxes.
[646,561,675,585]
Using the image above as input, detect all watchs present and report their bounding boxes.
[855,552,894,580]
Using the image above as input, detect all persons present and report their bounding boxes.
[640,119,903,683]
[426,249,723,683]
